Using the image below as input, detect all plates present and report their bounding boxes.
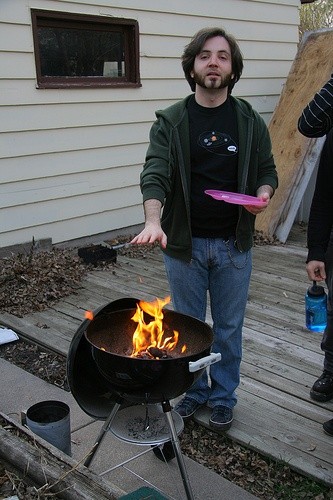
[204,190,266,205]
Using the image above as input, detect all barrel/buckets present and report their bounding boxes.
[26,400,72,458]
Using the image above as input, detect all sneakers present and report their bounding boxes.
[310,369,333,402]
[209,405,233,430]
[323,419,333,436]
[174,397,207,420]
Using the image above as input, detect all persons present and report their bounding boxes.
[130,26,278,432]
[297,75,333,438]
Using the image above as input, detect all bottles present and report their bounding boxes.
[304,280,328,332]
